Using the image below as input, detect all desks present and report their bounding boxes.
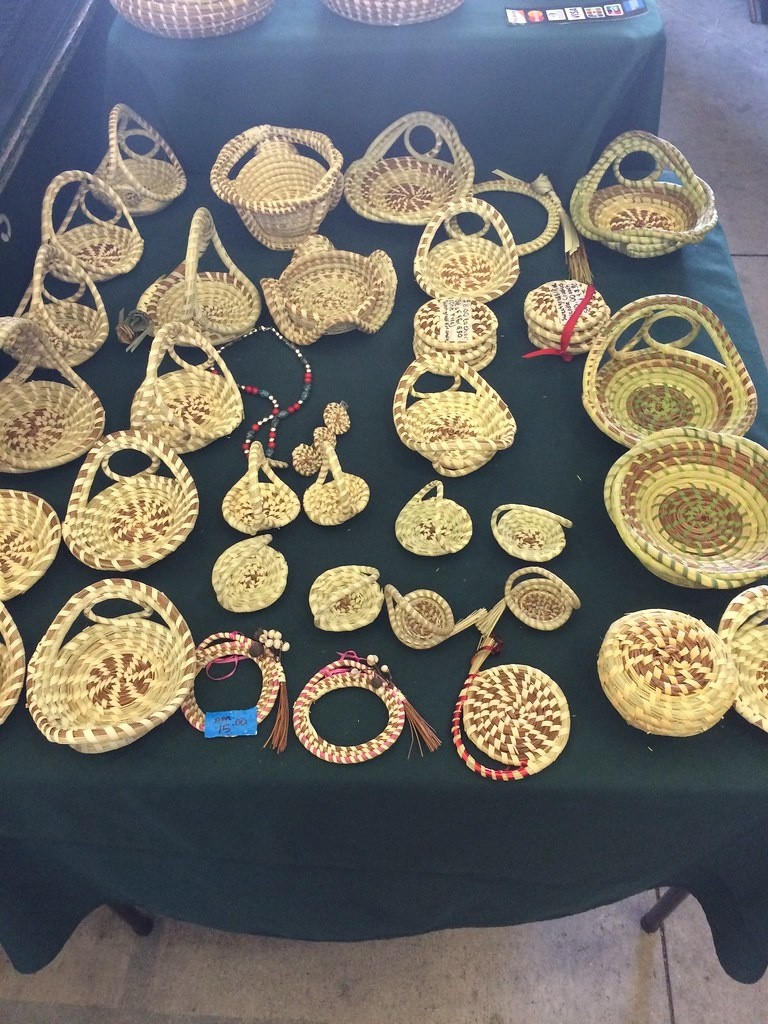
[0,0,768,986]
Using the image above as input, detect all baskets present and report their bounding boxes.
[322,0,461,26]
[0,100,579,781]
[579,293,768,739]
[570,129,721,259]
[109,0,277,38]
